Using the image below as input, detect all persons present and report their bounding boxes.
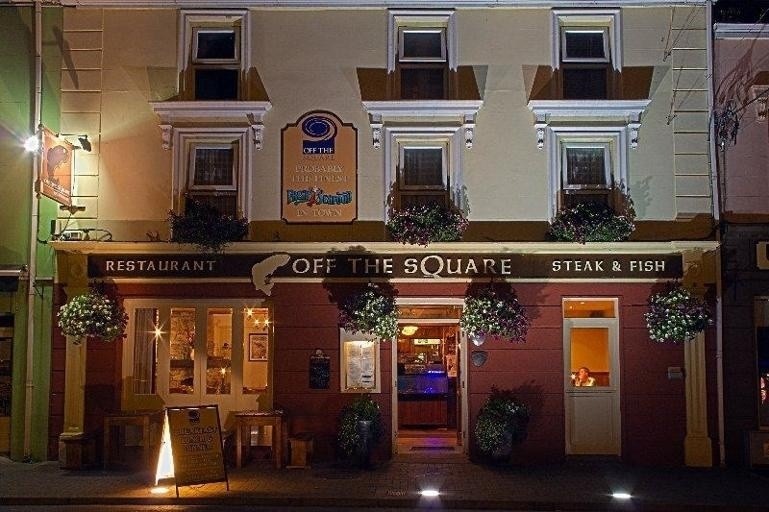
[576,367,598,387]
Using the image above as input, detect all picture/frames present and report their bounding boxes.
[249,333,268,361]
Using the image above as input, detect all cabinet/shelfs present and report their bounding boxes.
[399,373,450,427]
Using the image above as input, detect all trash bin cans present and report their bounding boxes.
[288,430,314,469]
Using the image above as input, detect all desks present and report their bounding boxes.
[104,414,157,473]
[235,411,286,473]
